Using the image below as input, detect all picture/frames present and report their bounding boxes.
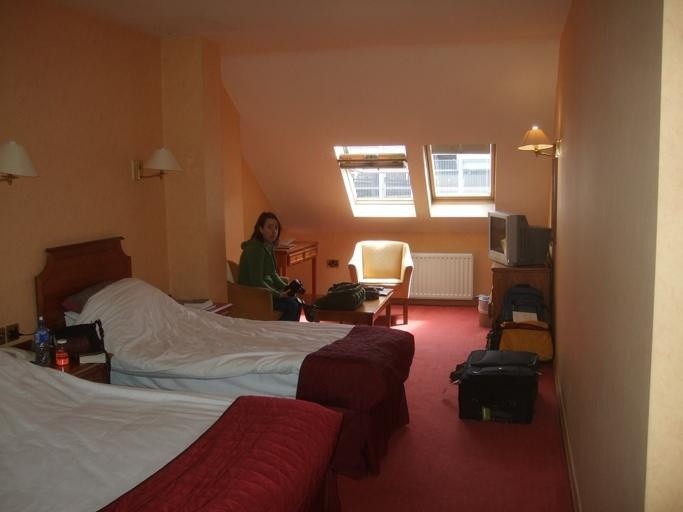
[273,241,319,300]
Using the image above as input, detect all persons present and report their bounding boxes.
[237,211,304,322]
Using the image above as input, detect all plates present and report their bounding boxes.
[491,261,552,327]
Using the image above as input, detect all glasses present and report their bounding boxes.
[518,126,561,160]
[131,149,183,184]
[0,141,39,186]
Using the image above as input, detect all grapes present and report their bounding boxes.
[174,300,234,317]
[10,340,114,383]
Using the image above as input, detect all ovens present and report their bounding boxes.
[0,349,342,512]
[34,236,414,480]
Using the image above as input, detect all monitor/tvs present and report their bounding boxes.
[79,351,107,364]
[379,288,394,296]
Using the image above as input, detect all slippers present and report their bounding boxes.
[502,284,544,321]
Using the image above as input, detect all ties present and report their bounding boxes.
[488,210,551,267]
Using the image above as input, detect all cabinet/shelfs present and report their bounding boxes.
[34,315,51,365]
[54,339,68,365]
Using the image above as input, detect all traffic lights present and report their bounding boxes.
[61,281,113,313]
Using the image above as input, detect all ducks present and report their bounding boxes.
[458,345,541,423]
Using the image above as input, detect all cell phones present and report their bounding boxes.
[284,278,305,297]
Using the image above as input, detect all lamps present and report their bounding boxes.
[226,260,240,283]
[348,240,414,324]
[226,281,285,321]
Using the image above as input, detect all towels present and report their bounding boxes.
[478,296,491,327]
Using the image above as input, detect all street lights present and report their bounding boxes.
[316,280,366,310]
[52,323,102,357]
[487,320,500,350]
[500,322,552,361]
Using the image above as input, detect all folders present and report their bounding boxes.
[408,253,474,300]
